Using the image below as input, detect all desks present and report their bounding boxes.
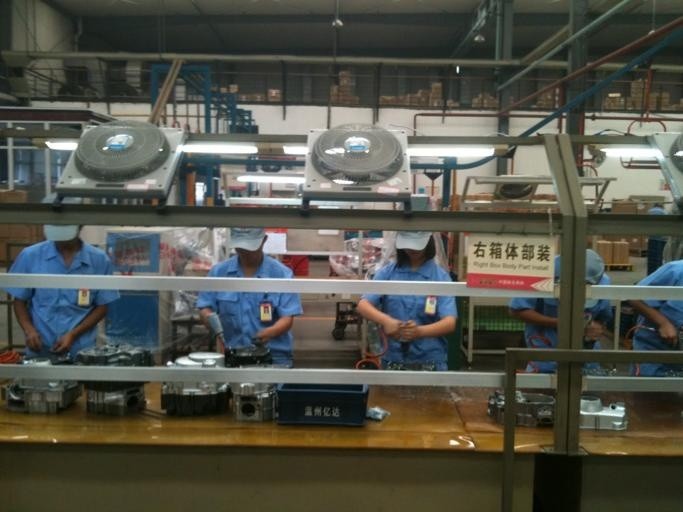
[0,381,683,511]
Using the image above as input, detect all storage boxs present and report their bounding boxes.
[602,79,682,113]
[535,89,559,110]
[211,84,283,103]
[276,384,369,427]
[379,83,446,110]
[592,196,665,265]
[471,93,498,109]
[331,70,360,106]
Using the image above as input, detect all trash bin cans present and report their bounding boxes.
[647,238,667,276]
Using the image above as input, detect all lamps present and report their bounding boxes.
[592,140,682,158]
[270,139,507,161]
[38,138,264,161]
[232,171,304,187]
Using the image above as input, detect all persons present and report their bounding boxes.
[5,191,123,368]
[626,258,682,376]
[353,230,459,371]
[644,203,669,242]
[195,226,308,369]
[508,246,613,375]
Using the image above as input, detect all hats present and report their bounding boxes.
[230,227,266,251]
[396,228,433,250]
[585,249,604,308]
[41,222,81,242]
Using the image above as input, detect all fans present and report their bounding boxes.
[52,119,187,213]
[303,123,413,213]
[651,132,682,211]
